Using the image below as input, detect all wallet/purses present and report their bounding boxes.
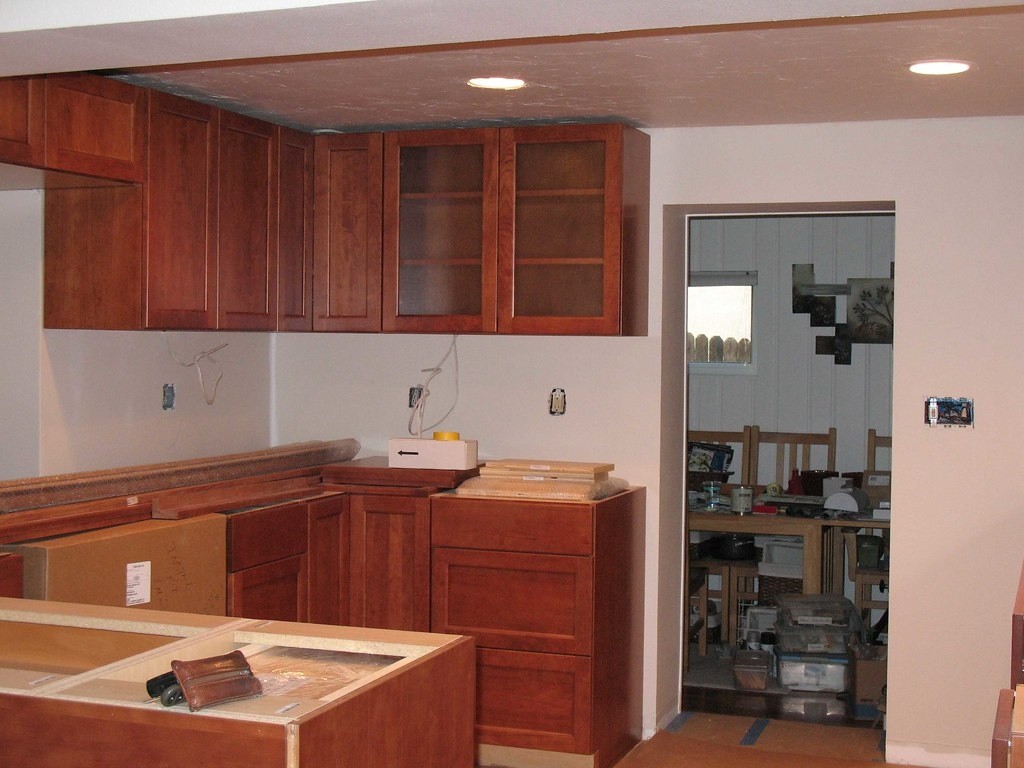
[171,650,263,712]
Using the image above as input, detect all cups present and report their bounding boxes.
[731,487,753,515]
[702,482,721,512]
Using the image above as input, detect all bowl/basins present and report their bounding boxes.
[802,470,838,495]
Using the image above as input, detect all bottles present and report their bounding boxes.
[697,334,708,363]
[724,337,737,362]
[760,631,775,653]
[687,333,696,362]
[746,631,760,651]
[737,338,751,363]
[709,336,724,362]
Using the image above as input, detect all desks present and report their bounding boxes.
[689,482,890,646]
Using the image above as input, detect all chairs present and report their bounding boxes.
[687,567,709,654]
[854,428,891,644]
[729,425,836,645]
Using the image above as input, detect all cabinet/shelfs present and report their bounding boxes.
[1,75,144,189]
[44,87,313,331]
[347,496,430,632]
[383,121,651,335]
[222,493,349,628]
[314,133,383,333]
[431,485,645,768]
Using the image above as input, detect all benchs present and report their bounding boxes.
[687,424,751,641]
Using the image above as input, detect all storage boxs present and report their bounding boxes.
[0,512,229,616]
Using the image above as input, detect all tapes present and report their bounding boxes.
[433,431,460,441]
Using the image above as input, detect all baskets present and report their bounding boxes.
[688,471,735,491]
[758,562,803,606]
